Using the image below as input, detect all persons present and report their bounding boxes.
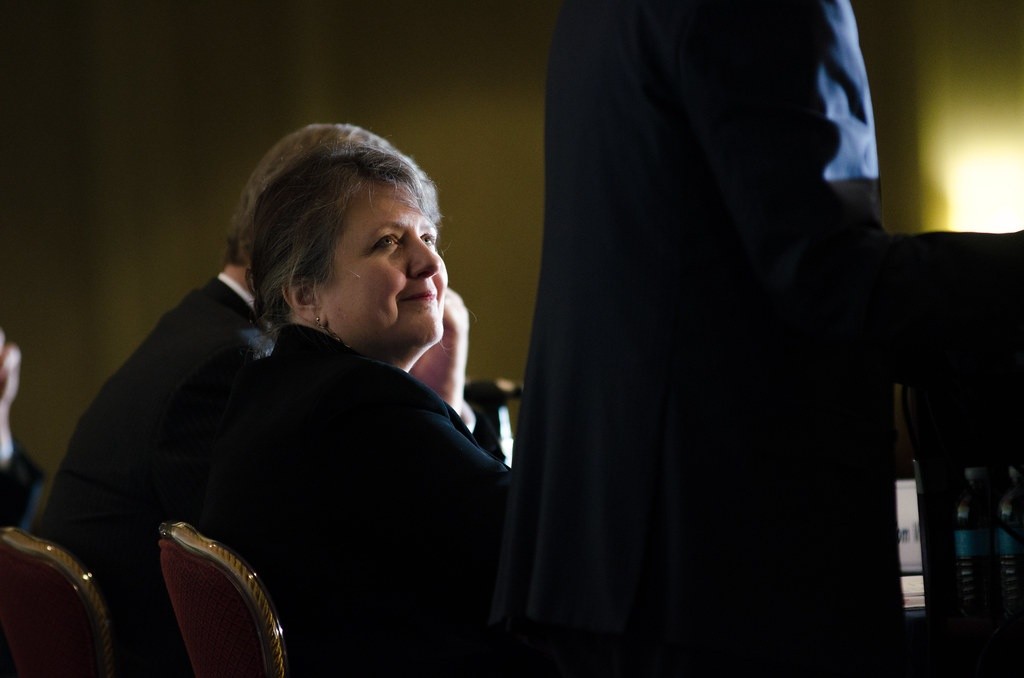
[198,141,512,678]
[39,121,504,678]
[0,330,49,678]
[487,0,1024,677]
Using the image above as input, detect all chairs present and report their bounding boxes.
[0,527,117,678]
[158,521,288,678]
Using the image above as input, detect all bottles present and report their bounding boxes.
[954,465,1024,636]
